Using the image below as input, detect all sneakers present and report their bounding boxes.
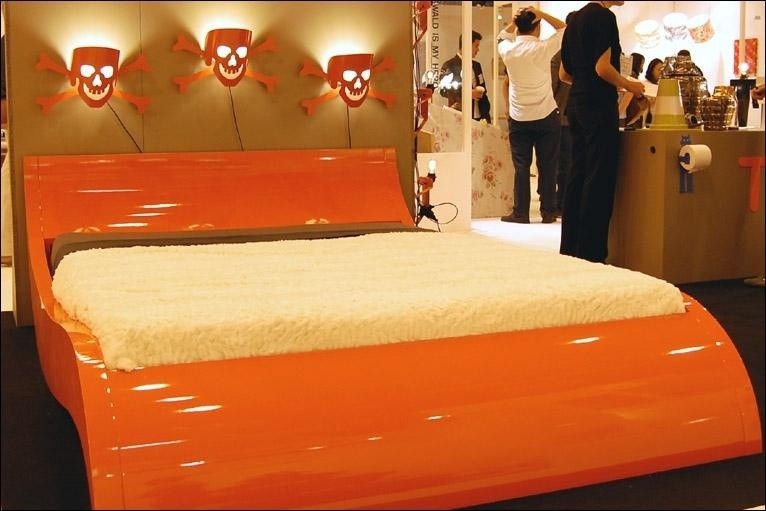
[744,274,765,286]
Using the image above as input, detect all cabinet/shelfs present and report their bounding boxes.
[604,127,766,285]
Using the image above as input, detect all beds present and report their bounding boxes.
[20,147,766,511]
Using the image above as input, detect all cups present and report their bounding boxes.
[474,87,485,101]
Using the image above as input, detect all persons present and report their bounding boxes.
[551,48,568,217]
[643,58,664,97]
[678,50,704,77]
[744,84,766,287]
[628,52,645,79]
[497,6,567,224]
[440,31,491,124]
[559,0,644,264]
[502,68,509,121]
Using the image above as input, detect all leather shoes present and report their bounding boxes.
[501,210,529,223]
[542,214,556,223]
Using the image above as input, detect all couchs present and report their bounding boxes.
[430,105,559,218]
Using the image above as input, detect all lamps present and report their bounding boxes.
[736,61,750,79]
[35,50,153,116]
[417,71,439,130]
[170,30,279,94]
[298,53,398,117]
[415,159,441,231]
[633,0,716,49]
